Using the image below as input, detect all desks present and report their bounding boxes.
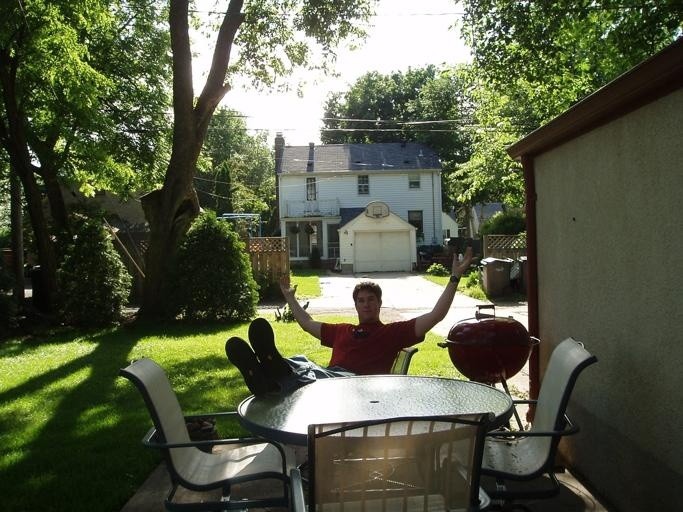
[238,375,516,450]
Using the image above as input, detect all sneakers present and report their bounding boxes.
[225,337,274,397]
[249,318,293,383]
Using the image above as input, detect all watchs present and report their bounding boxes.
[450,275,461,283]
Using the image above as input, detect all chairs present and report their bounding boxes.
[297,346,419,484]
[117,355,298,512]
[484,335,598,512]
[289,411,492,512]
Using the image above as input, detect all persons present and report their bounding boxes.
[226,245,473,397]
